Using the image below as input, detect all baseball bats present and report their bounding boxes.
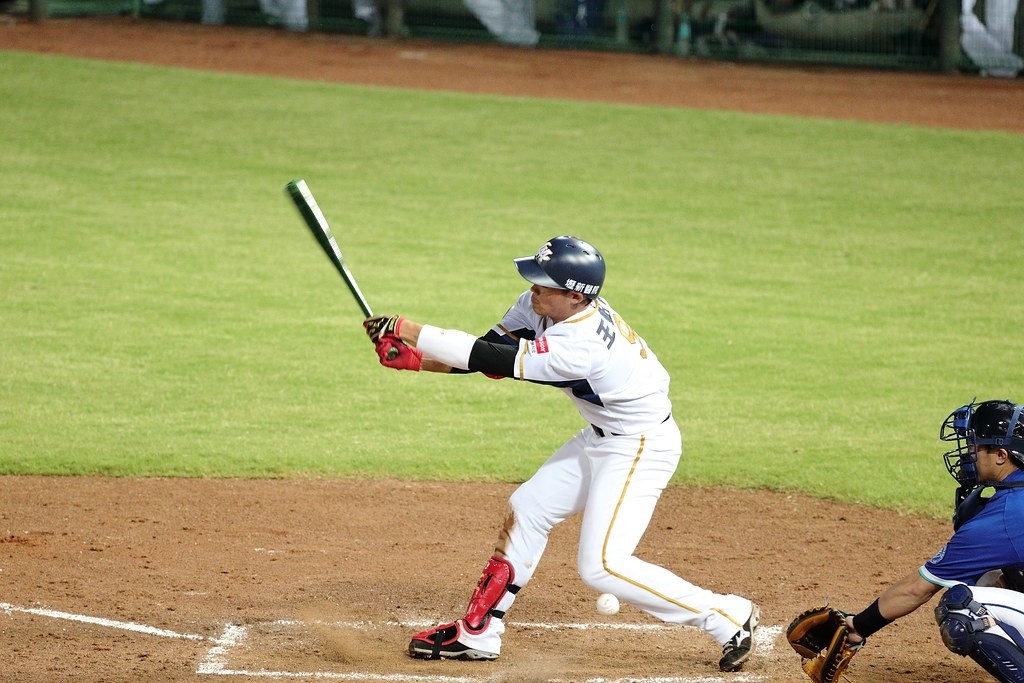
[285,176,401,361]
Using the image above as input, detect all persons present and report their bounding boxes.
[787,396,1024,683]
[363,233,762,672]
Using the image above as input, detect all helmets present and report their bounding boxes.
[513,235,606,300]
[940,397,1024,512]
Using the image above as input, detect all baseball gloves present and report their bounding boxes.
[786,605,867,683]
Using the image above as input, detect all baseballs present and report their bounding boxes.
[595,592,620,616]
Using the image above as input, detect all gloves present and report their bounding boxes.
[375,336,423,372]
[362,313,404,344]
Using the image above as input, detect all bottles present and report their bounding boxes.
[576,0,588,29]
[616,1,629,43]
[677,13,692,54]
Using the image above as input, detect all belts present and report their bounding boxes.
[591,413,671,437]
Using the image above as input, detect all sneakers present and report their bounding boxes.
[719,601,761,672]
[407,626,500,660]
